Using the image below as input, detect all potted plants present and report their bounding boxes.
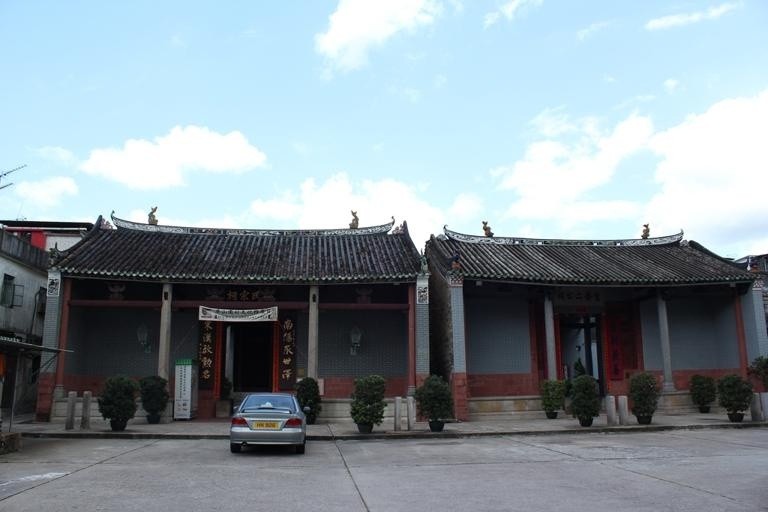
[98,375,170,431]
[540,356,768,427]
[295,374,454,434]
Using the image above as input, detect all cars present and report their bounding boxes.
[230,392,311,455]
